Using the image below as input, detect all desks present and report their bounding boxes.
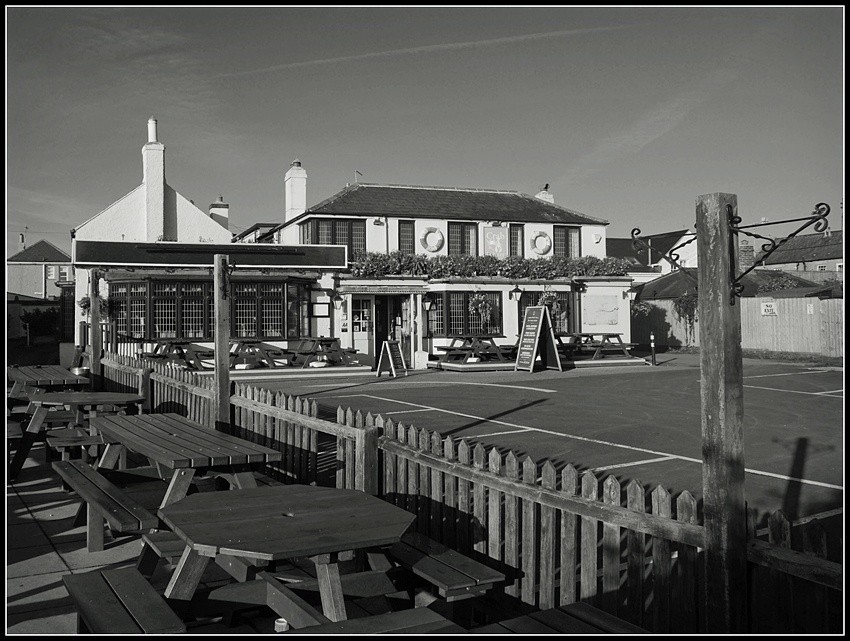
[90,412,282,571]
[154,338,203,371]
[449,334,506,365]
[225,336,274,369]
[555,332,633,361]
[7,365,90,427]
[9,391,148,484]
[155,485,416,624]
[295,337,349,368]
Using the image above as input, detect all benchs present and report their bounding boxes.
[6,388,506,634]
[434,343,519,366]
[555,339,639,362]
[138,332,358,369]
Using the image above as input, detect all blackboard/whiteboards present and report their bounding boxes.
[516,306,561,370]
[378,341,407,371]
